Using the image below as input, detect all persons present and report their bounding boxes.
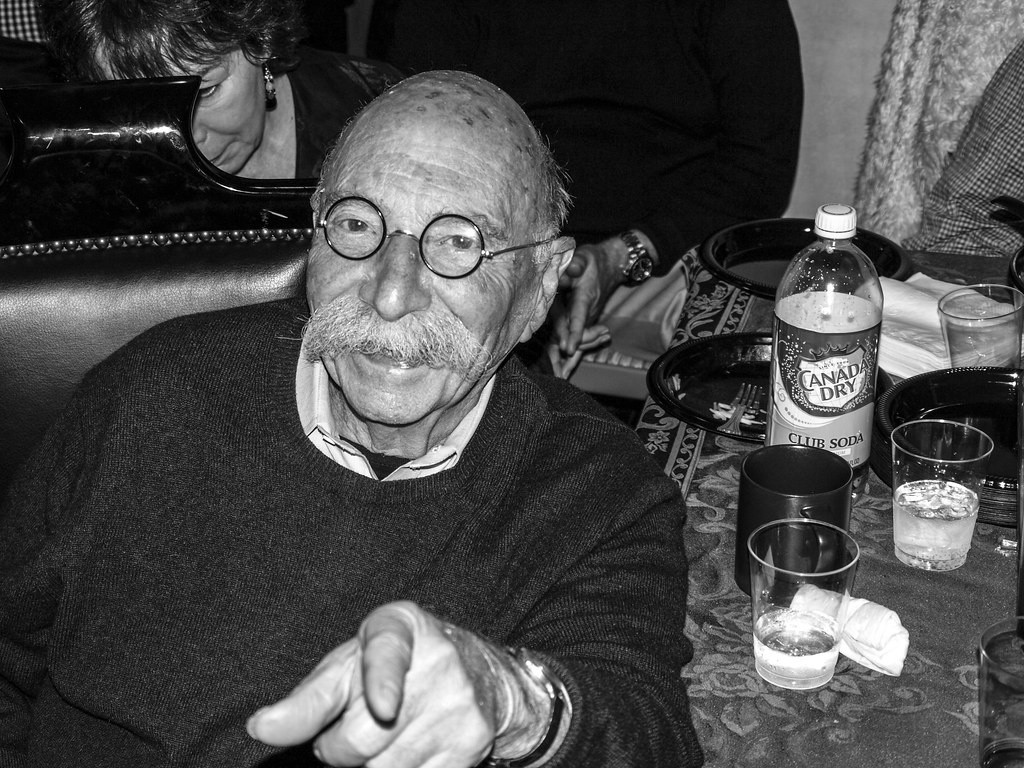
[30,0,805,381]
[0,67,705,767]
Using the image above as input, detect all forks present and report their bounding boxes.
[717,383,762,436]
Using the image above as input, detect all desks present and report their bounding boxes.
[637,249,1024,768]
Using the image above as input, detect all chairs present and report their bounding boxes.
[0,75,323,480]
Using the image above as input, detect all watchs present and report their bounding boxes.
[487,644,566,768]
[620,227,655,288]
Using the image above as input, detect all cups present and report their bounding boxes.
[938,284,1024,370]
[891,419,993,571]
[747,519,859,690]
[980,616,1024,768]
[733,444,853,607]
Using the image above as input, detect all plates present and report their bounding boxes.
[696,218,910,301]
[647,331,894,443]
[871,366,1024,529]
[1011,247,1024,293]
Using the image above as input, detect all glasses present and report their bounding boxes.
[314,194,562,280]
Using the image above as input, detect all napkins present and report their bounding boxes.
[858,273,1024,378]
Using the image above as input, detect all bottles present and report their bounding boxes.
[767,204,883,509]
